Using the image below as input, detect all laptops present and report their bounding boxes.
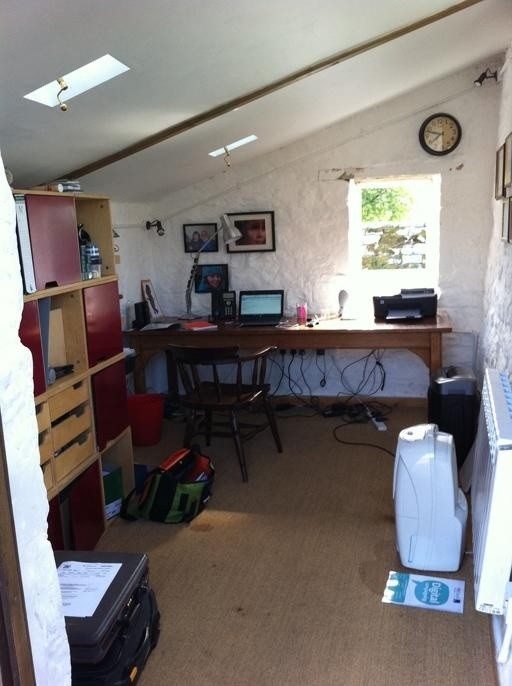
[238,290,284,326]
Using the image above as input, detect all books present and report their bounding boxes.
[183,321,218,331]
[194,471,208,482]
[80,245,91,281]
[50,180,84,192]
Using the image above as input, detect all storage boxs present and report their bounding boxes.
[101,460,125,520]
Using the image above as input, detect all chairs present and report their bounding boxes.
[165,343,283,483]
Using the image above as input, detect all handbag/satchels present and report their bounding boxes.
[119,443,217,526]
[50,549,152,667]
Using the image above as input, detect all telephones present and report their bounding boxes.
[211,288,237,323]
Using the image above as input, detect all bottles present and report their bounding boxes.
[86,245,102,277]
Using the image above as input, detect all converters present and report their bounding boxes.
[316,349,326,355]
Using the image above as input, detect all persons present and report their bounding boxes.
[198,266,226,291]
[188,231,203,251]
[242,221,266,244]
[200,231,217,250]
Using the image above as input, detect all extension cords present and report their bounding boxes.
[366,406,388,432]
[278,348,311,358]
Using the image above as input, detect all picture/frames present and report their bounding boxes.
[195,263,229,293]
[494,132,512,243]
[224,211,276,253]
[183,222,219,252]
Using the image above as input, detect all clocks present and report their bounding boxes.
[419,112,462,157]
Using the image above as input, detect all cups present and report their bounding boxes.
[296,302,308,323]
[321,309,330,320]
[134,301,150,328]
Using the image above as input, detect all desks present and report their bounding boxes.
[122,311,453,394]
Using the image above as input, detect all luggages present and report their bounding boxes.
[72,584,164,686]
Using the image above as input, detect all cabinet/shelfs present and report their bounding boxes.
[11,188,137,552]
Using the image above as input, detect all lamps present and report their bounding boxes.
[474,67,498,88]
[146,219,164,236]
[178,213,243,320]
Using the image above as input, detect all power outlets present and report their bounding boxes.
[279,348,307,356]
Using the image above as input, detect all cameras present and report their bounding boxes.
[373,288,437,322]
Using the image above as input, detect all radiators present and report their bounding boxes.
[470,369,511,616]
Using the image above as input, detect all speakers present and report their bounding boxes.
[135,302,150,324]
